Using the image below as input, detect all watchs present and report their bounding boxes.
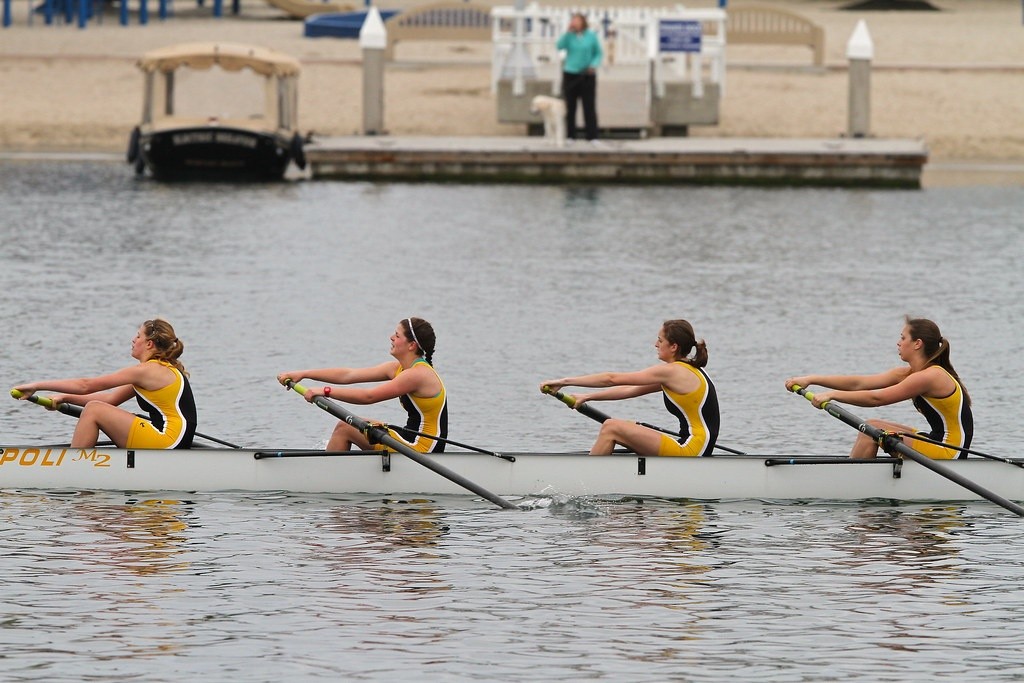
[323,386,331,397]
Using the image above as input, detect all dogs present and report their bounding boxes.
[529,95,567,146]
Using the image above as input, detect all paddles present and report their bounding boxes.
[542,384,612,424]
[790,384,1024,519]
[283,377,522,511]
[9,388,215,449]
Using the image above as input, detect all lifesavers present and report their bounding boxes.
[127,125,141,162]
[290,130,306,170]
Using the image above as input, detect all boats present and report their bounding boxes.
[127,43,306,184]
[0,440,1024,502]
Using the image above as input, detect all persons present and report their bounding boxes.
[279,317,448,454]
[540,319,720,457]
[14,319,197,451]
[784,319,974,460]
[556,14,603,140]
